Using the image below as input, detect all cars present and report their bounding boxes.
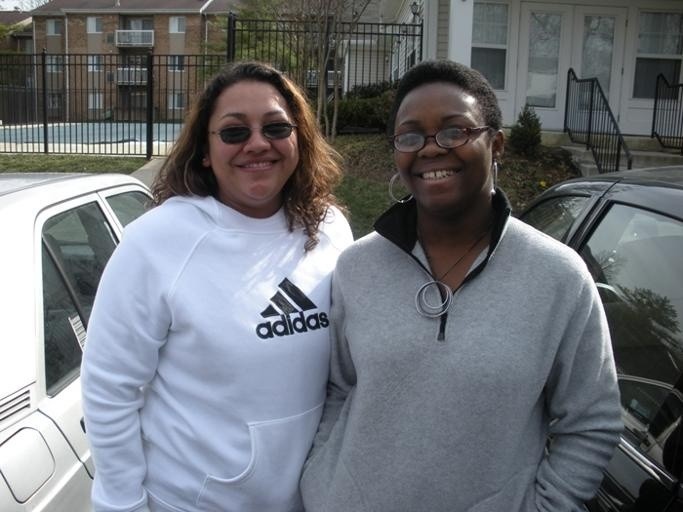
[0,167,163,512]
[512,161,683,512]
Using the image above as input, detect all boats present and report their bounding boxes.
[615,372,681,472]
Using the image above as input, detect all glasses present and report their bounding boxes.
[390,126,486,153]
[204,122,299,144]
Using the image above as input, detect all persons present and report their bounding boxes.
[293,59,624,512]
[71,58,357,510]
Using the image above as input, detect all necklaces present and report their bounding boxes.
[402,228,499,320]
